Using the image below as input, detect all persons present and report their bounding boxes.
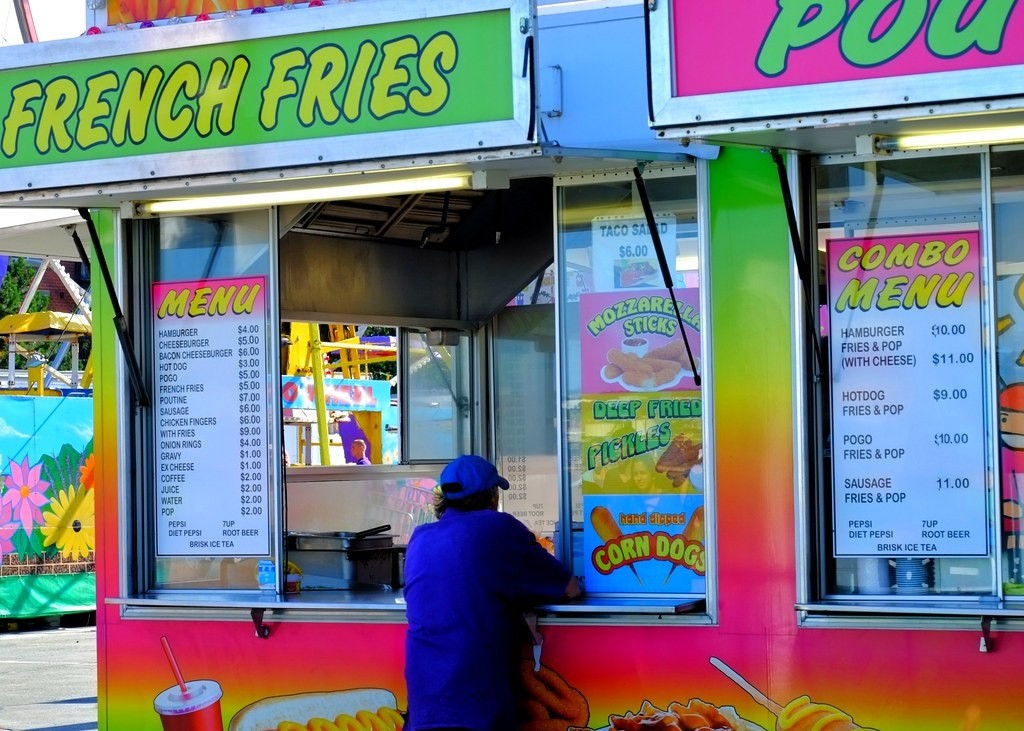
[403,455,580,730]
[350,439,372,466]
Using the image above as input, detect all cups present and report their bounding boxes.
[856,559,891,594]
[621,337,648,357]
[286,574,302,593]
[153,679,224,731]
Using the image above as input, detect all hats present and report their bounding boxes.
[440,455,510,498]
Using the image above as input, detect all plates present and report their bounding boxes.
[895,559,929,588]
